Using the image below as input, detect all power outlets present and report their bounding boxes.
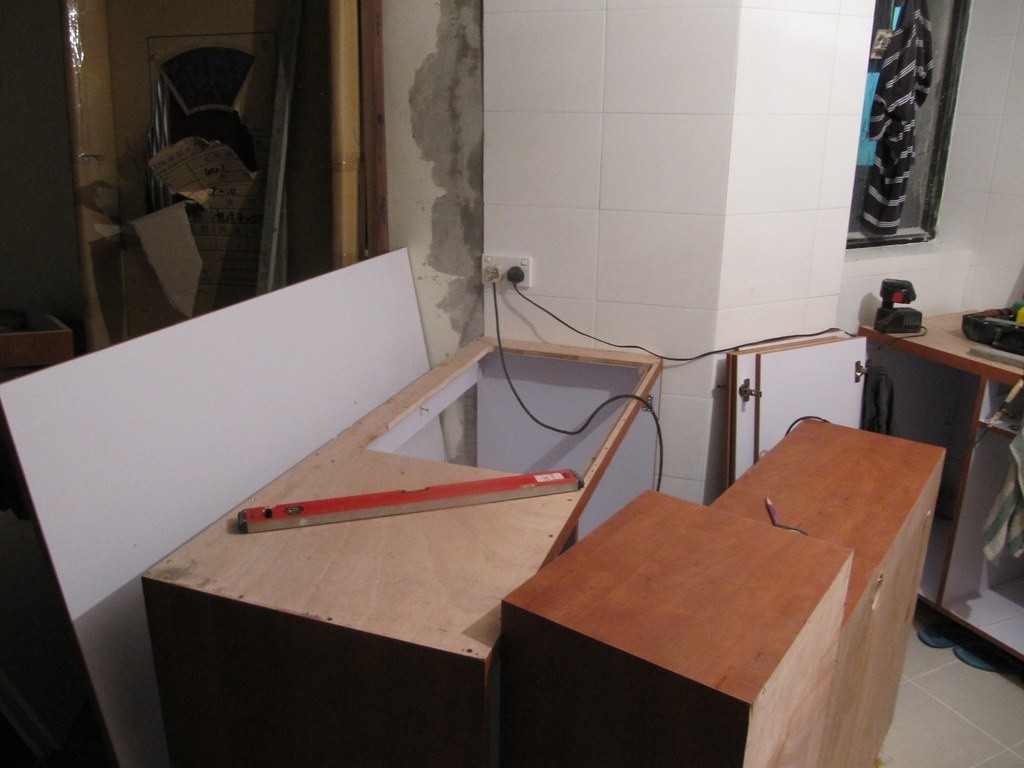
[481,253,532,287]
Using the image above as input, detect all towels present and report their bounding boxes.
[979,427,1024,569]
[861,0,934,236]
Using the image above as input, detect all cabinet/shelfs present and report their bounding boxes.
[144,521,577,768]
[726,309,1024,662]
[500,418,946,768]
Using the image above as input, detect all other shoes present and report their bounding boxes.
[953,642,1024,672]
[919,621,978,648]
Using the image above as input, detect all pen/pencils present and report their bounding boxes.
[765,495,780,527]
